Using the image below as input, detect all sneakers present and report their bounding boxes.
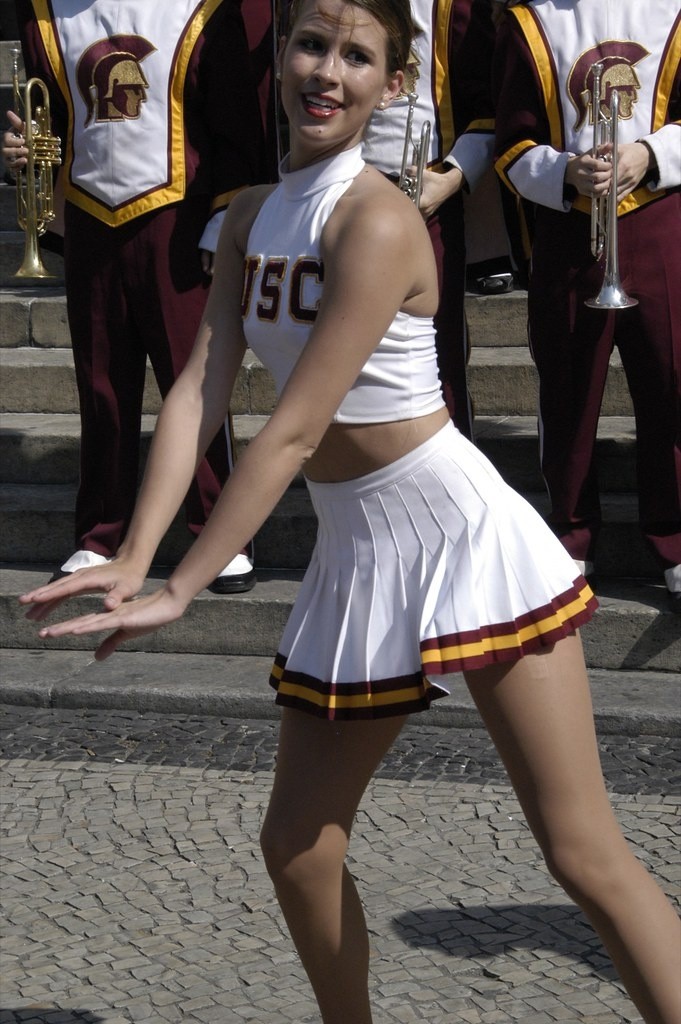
[211,555,257,592]
[53,550,118,583]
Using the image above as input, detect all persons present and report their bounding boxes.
[1,0,681,593]
[15,0,681,1024]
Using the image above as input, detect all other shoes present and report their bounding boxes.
[572,559,595,584]
[664,563,681,614]
[474,273,514,292]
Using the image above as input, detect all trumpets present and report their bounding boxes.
[9,47,63,281]
[396,90,431,210]
[584,62,639,312]
